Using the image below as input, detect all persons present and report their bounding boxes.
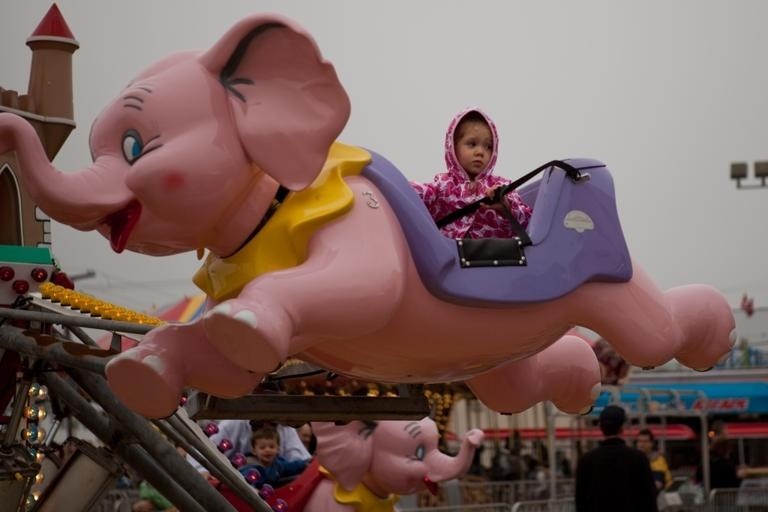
[575,405,657,511]
[130,443,188,511]
[694,422,741,495]
[234,427,313,490]
[186,420,313,481]
[297,422,313,449]
[636,428,673,490]
[470,429,572,480]
[408,107,534,240]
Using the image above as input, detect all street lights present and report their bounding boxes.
[728,160,768,191]
